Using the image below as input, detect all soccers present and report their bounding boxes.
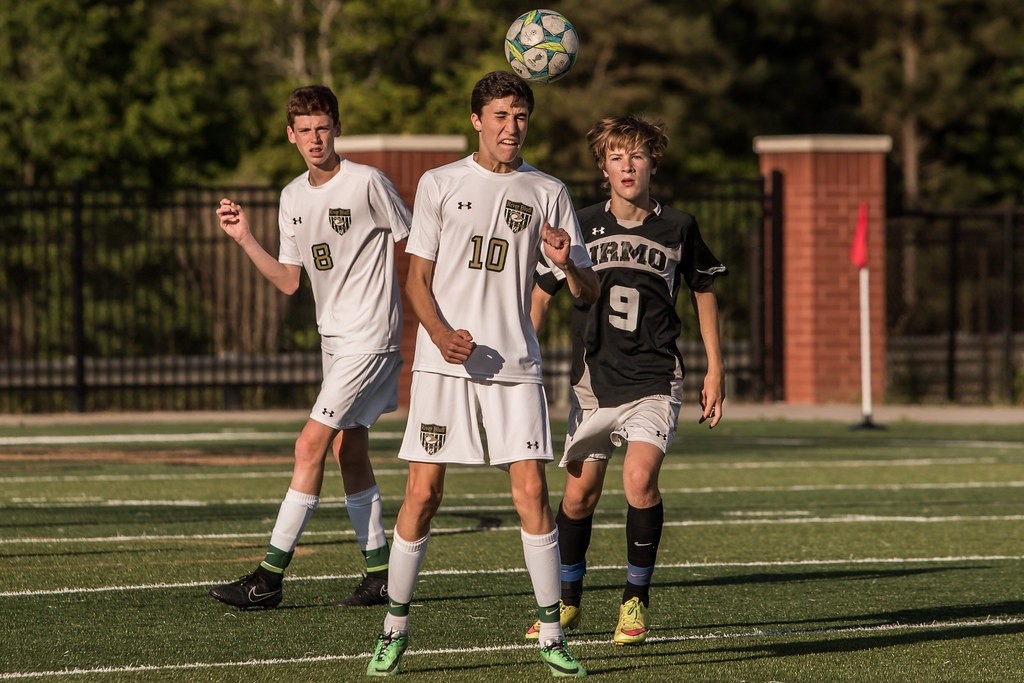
[502,6,580,86]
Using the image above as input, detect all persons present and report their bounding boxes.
[368,71,600,677]
[210,84,412,609]
[524,114,725,649]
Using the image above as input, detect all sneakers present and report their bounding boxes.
[613,595,648,645]
[209,564,284,610]
[539,640,587,678]
[525,598,583,641]
[336,572,388,607]
[366,627,408,677]
